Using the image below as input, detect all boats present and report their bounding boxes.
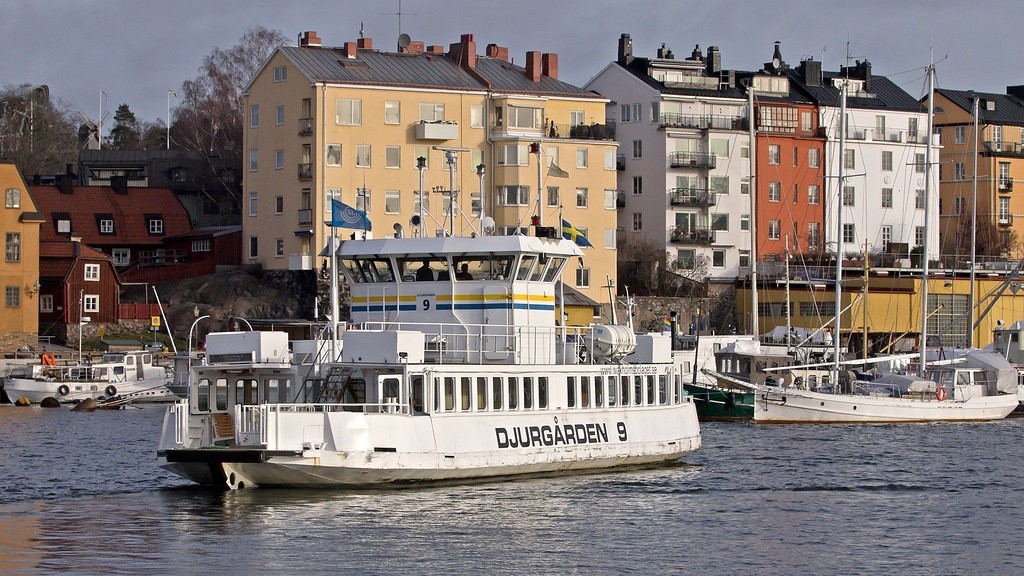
[156,152,705,489]
[3,288,171,408]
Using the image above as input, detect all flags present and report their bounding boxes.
[662,314,672,330]
[562,218,594,248]
[332,198,372,230]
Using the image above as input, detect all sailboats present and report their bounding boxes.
[682,44,1024,423]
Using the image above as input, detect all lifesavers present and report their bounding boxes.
[59,384,70,396]
[936,387,947,401]
[106,385,117,396]
